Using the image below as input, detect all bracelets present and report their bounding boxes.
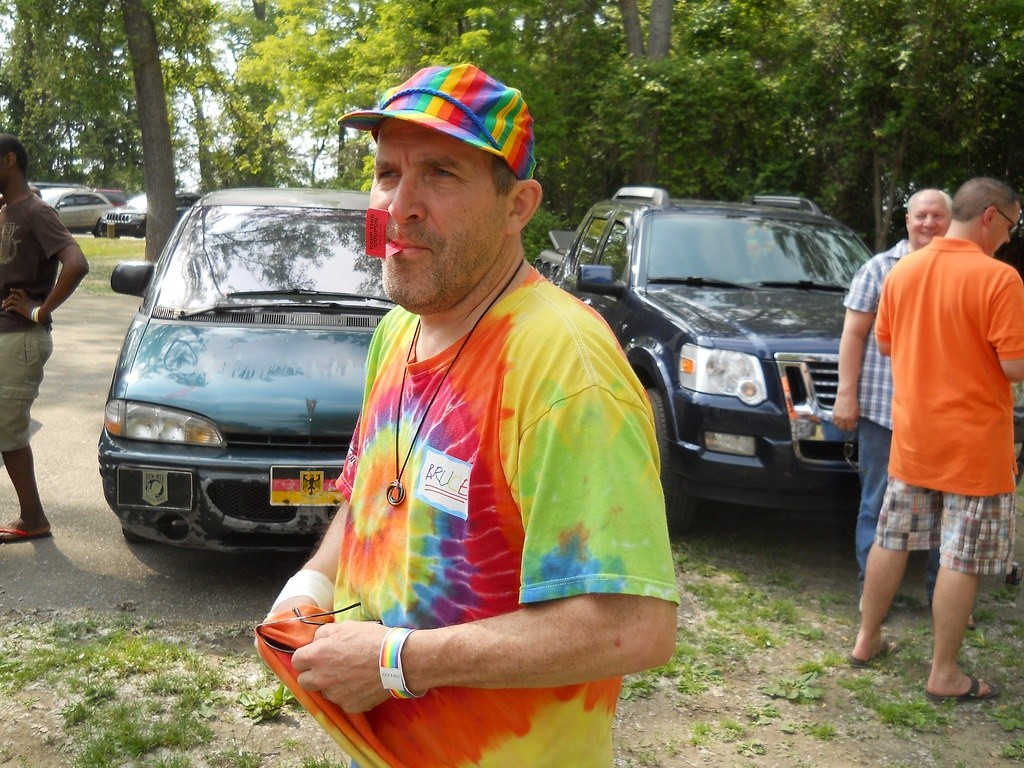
[34,307,41,323]
[378,627,422,700]
[272,567,335,612]
[31,308,36,321]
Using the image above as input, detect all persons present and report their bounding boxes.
[831,188,953,623]
[847,176,1024,703]
[0,132,90,544]
[253,61,681,767]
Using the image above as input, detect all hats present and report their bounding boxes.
[336,63,536,180]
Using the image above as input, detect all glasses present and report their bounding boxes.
[984,207,1018,234]
[254,601,383,654]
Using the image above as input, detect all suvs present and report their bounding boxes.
[551,186,877,510]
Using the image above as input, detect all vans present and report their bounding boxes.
[92,187,126,208]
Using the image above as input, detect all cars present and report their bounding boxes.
[39,187,114,238]
[27,182,90,193]
[98,186,399,553]
[100,191,201,240]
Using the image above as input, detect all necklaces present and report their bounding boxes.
[385,258,527,509]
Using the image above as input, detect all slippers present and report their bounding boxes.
[923,674,999,701]
[0,528,52,543]
[851,639,901,668]
